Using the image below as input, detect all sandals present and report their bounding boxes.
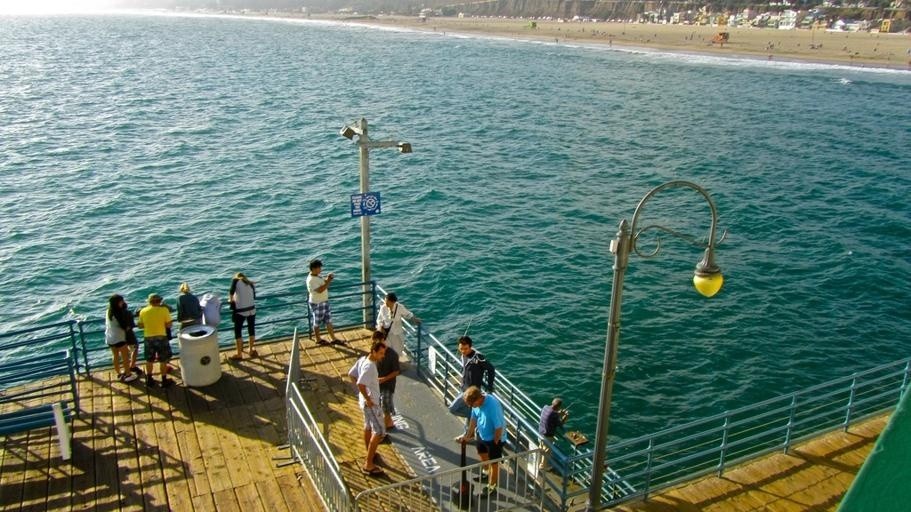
[360,465,385,475]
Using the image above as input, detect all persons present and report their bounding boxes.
[305,259,346,344]
[227,272,257,359]
[104,295,138,382]
[199,292,220,327]
[376,289,422,359]
[123,303,143,374]
[455,386,508,498]
[137,292,172,387]
[372,330,401,430]
[538,397,568,469]
[177,283,199,331]
[449,336,495,442]
[135,302,173,360]
[348,341,387,474]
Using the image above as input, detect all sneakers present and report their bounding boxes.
[129,366,138,371]
[161,379,172,385]
[115,373,139,383]
[472,474,489,485]
[145,380,159,387]
[454,433,475,443]
[538,464,553,473]
[477,486,497,500]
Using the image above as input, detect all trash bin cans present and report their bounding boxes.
[176,325,222,387]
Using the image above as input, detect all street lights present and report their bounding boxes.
[583,177,728,512]
[340,112,414,330]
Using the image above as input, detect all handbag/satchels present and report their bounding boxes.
[381,303,399,341]
[232,310,238,323]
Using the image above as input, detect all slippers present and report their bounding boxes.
[229,354,242,361]
[331,340,345,346]
[315,339,330,346]
[248,350,258,358]
